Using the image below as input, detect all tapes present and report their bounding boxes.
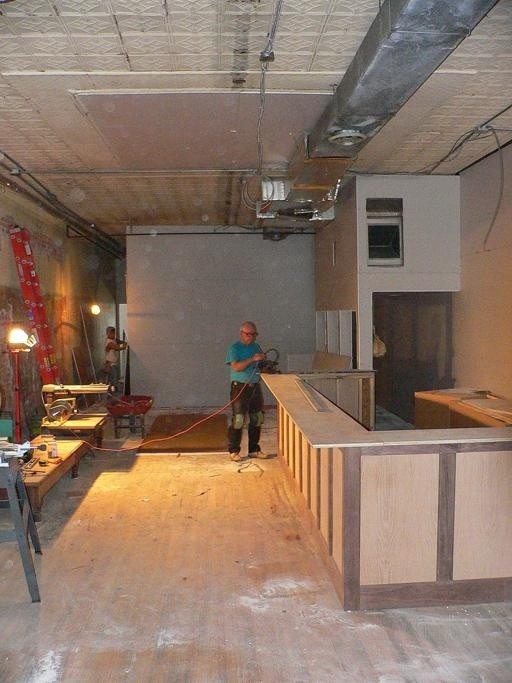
[39,460,46,465]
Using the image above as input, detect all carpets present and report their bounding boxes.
[133,414,230,454]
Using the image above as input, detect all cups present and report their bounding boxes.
[47,442,57,458]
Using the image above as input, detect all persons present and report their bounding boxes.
[224,321,275,461]
[104,326,127,385]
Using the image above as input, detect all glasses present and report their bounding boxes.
[242,330,259,337]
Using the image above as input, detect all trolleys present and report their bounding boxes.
[105,396,154,437]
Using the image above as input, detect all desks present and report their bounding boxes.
[0,412,112,523]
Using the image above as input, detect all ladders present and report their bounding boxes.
[9,227,62,395]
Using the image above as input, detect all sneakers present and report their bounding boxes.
[230,453,243,462]
[248,451,269,459]
